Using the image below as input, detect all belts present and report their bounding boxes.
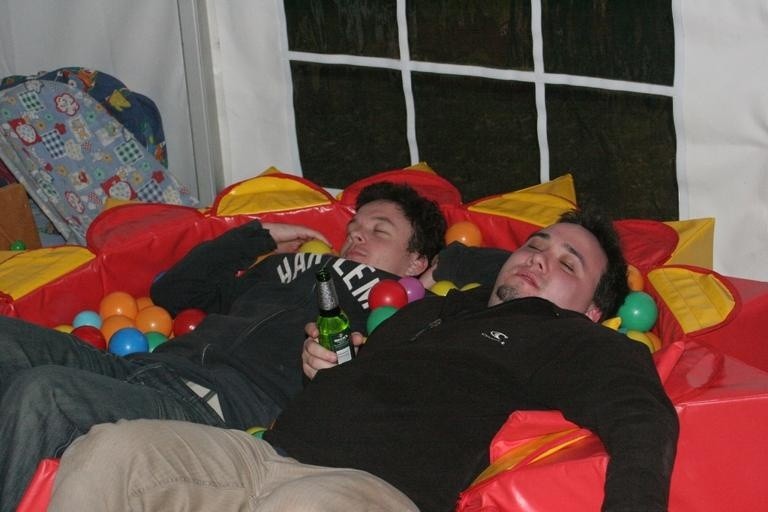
[179,374,225,423]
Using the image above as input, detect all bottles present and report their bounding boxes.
[313,268,355,367]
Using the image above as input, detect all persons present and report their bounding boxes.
[0,178,451,511]
[39,202,683,511]
